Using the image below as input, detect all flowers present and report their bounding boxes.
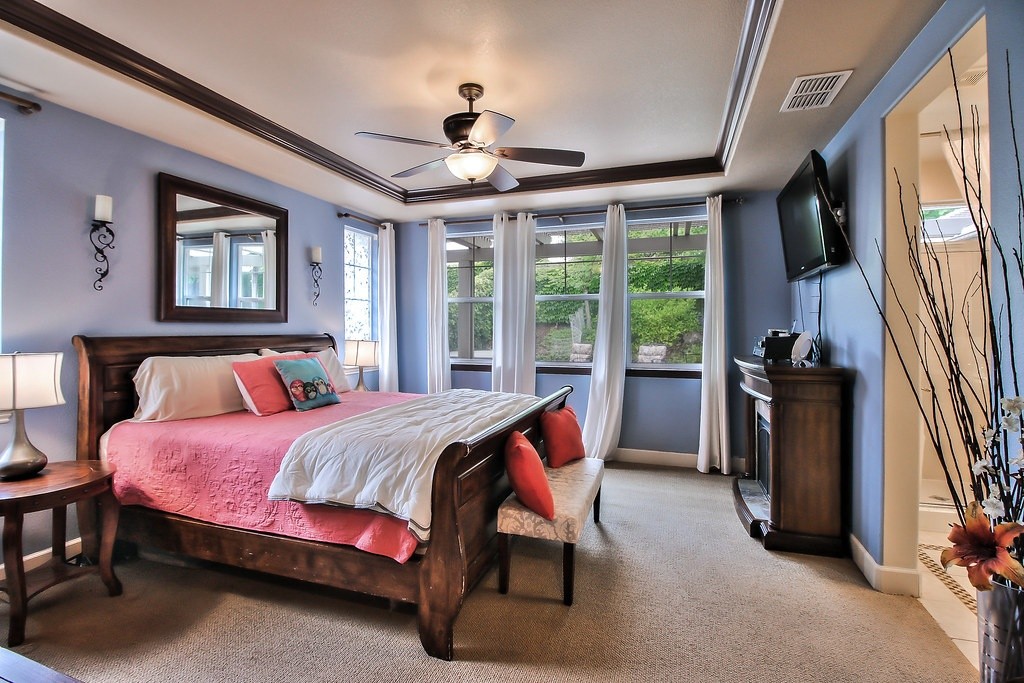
[817,48,1024,596]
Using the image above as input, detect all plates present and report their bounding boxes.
[791,331,812,363]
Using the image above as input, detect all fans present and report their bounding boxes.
[355,83,586,192]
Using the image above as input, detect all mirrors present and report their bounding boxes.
[157,170,288,324]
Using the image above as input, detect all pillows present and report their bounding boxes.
[231,353,337,417]
[539,405,585,467]
[272,356,342,412]
[131,354,258,423]
[505,430,554,522]
[243,347,351,413]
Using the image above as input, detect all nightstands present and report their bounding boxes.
[0,458,122,646]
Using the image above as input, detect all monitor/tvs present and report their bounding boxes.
[777,148,849,282]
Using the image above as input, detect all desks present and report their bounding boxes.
[733,355,848,555]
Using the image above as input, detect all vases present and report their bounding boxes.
[976,582,1024,683]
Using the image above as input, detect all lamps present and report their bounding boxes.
[0,349,66,480]
[343,339,380,392]
[444,148,498,190]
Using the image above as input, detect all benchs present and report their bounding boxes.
[495,456,605,605]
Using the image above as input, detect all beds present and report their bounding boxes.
[71,333,574,663]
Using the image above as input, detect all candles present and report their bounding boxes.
[94,194,113,220]
[310,246,322,262]
